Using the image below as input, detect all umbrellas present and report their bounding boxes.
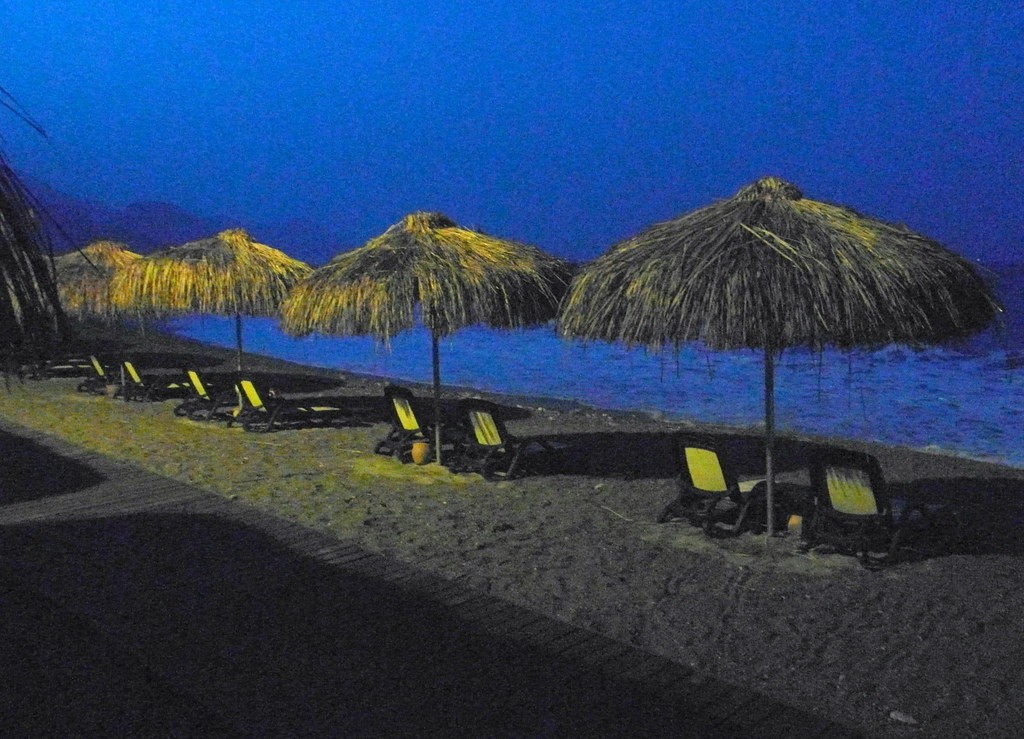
[555,176,1007,536]
[279,211,579,464]
[10,228,321,370]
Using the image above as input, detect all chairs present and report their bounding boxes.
[655,442,780,534]
[373,384,460,463]
[470,406,571,481]
[797,452,956,556]
[78,341,117,394]
[123,350,213,401]
[175,355,269,419]
[240,378,345,432]
